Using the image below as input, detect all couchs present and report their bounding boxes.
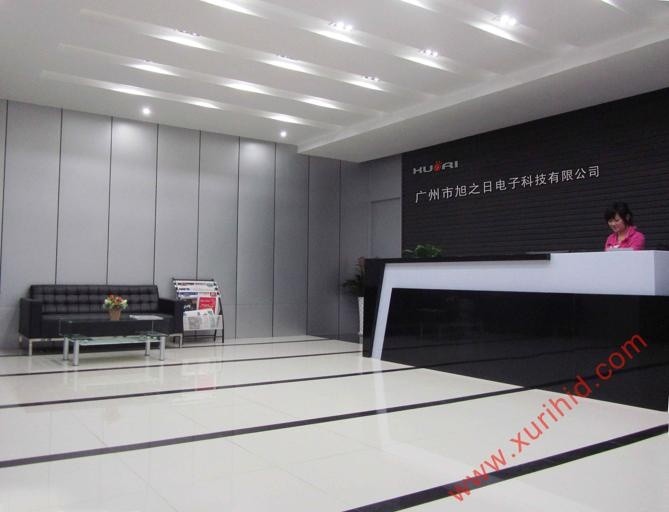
[19,285,184,357]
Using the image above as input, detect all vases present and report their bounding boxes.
[108,307,120,321]
[357,295,366,335]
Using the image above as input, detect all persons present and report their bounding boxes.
[602,201,647,250]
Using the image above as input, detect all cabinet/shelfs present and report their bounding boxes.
[173,278,224,343]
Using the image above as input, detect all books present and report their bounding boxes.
[172,280,221,332]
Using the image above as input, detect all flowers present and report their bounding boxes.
[100,291,129,312]
[342,255,366,298]
